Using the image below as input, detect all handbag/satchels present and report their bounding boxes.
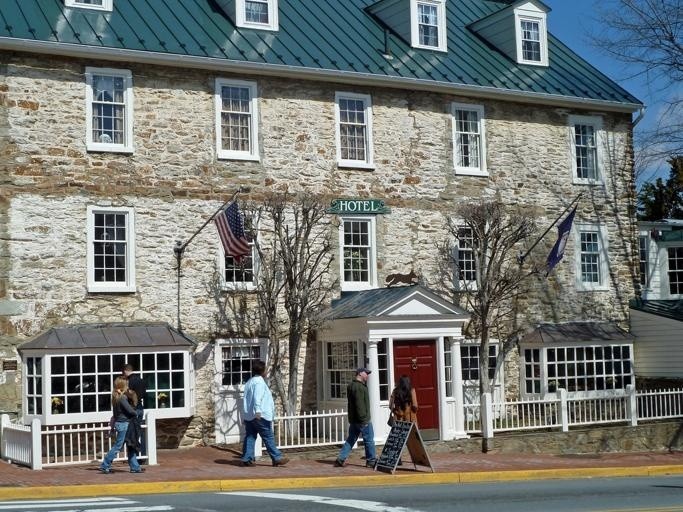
[387,414,394,427]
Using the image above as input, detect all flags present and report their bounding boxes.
[546,204,578,276]
[213,199,251,264]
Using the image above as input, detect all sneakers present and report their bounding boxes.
[365,458,378,468]
[239,460,257,467]
[273,455,290,466]
[129,466,146,473]
[334,459,350,467]
[101,466,116,474]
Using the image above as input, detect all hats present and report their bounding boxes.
[356,367,372,375]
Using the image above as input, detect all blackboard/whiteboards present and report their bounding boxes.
[376,420,413,469]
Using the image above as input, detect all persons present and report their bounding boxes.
[335,367,378,468]
[389,375,419,423]
[98,375,146,475]
[121,364,146,466]
[240,361,290,467]
